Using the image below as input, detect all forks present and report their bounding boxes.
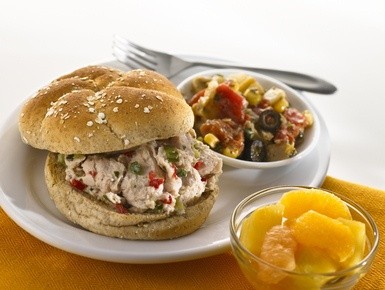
[111,34,338,95]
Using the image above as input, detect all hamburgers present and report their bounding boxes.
[16,64,225,240]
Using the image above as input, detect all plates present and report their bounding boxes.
[175,69,322,169]
[0,53,331,262]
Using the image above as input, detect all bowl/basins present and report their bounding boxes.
[230,185,381,286]
